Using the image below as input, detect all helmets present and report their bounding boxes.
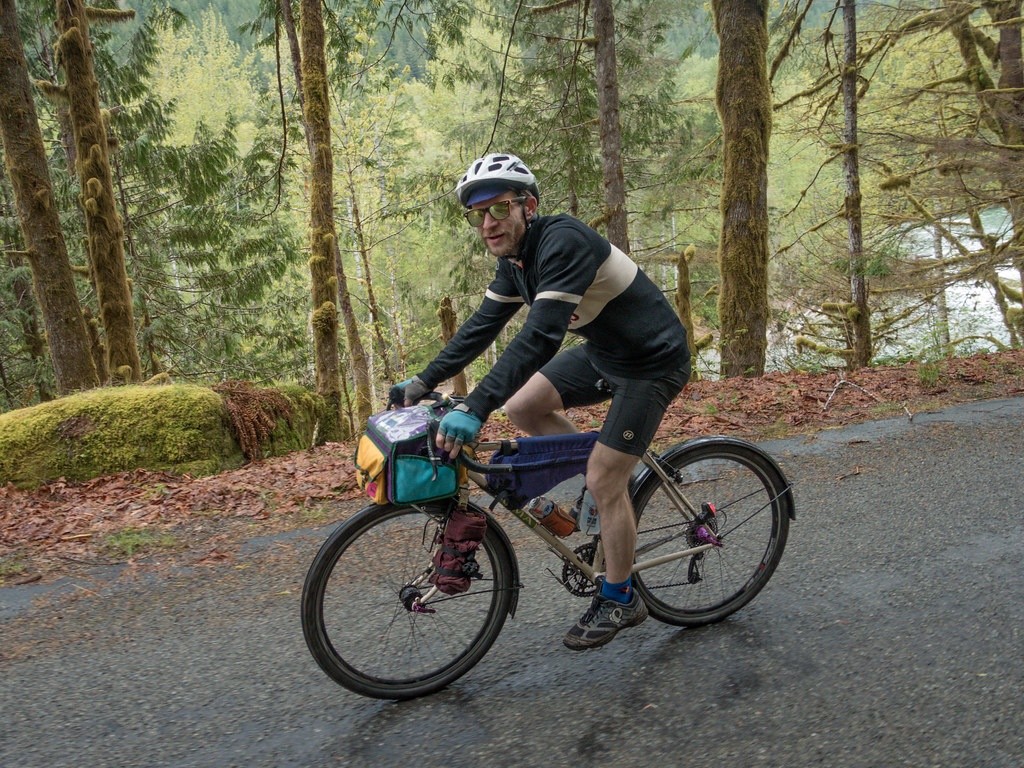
[455,153,540,207]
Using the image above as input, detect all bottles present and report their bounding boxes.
[529,496,575,537]
[580,490,600,535]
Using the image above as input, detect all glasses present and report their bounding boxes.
[463,197,527,227]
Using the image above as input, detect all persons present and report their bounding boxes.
[389,153,691,651]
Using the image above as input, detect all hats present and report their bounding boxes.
[464,185,511,207]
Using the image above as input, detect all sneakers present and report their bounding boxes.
[563,582,650,651]
[567,488,588,534]
